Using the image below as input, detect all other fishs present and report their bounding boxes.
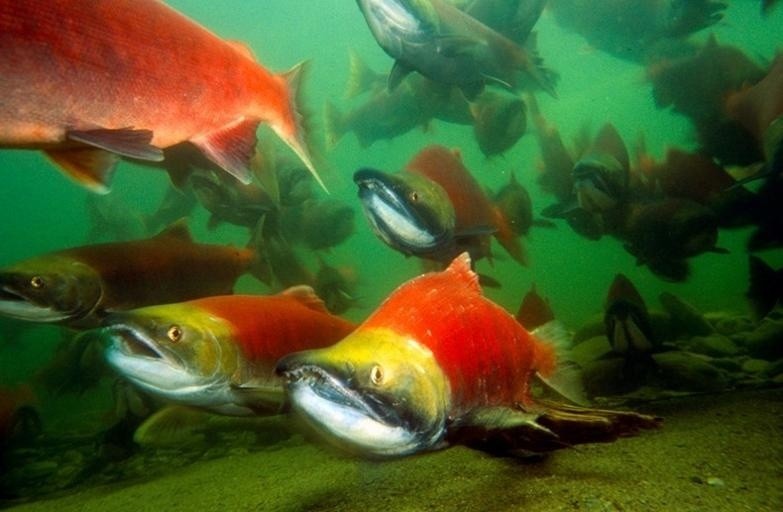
[1,0,782,467]
